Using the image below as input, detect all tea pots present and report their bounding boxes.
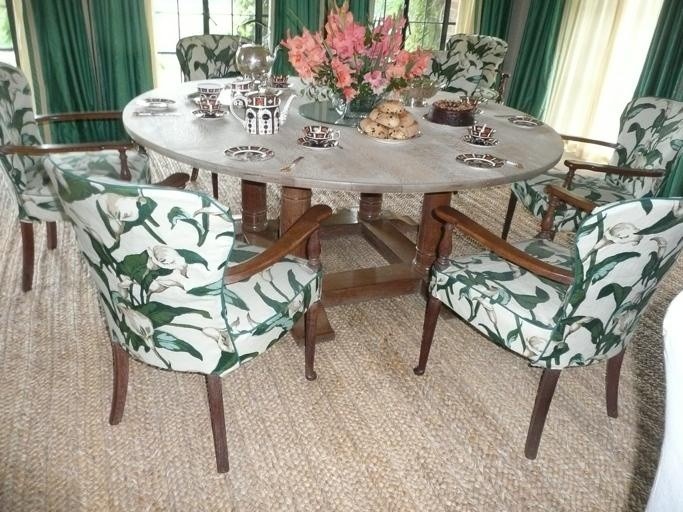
[229,87,297,136]
[233,19,283,81]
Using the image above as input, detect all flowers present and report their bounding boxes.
[276,0,437,114]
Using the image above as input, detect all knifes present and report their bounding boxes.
[494,115,526,118]
[134,113,178,117]
[280,156,303,171]
[489,156,522,167]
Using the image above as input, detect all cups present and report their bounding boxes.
[272,76,287,84]
[467,126,496,142]
[302,126,340,143]
[199,100,220,115]
[197,83,222,99]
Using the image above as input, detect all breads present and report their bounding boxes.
[359,99,420,141]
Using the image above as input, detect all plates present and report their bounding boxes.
[139,98,175,110]
[187,92,220,106]
[459,95,489,104]
[422,113,479,128]
[224,146,273,163]
[296,137,339,150]
[354,122,423,146]
[461,135,499,150]
[192,109,228,121]
[507,117,544,129]
[268,83,292,90]
[457,153,503,172]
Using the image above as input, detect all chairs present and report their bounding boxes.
[176,34,258,200]
[44,155,333,473]
[0,63,152,292]
[413,184,683,460]
[432,33,509,195]
[501,95,683,245]
